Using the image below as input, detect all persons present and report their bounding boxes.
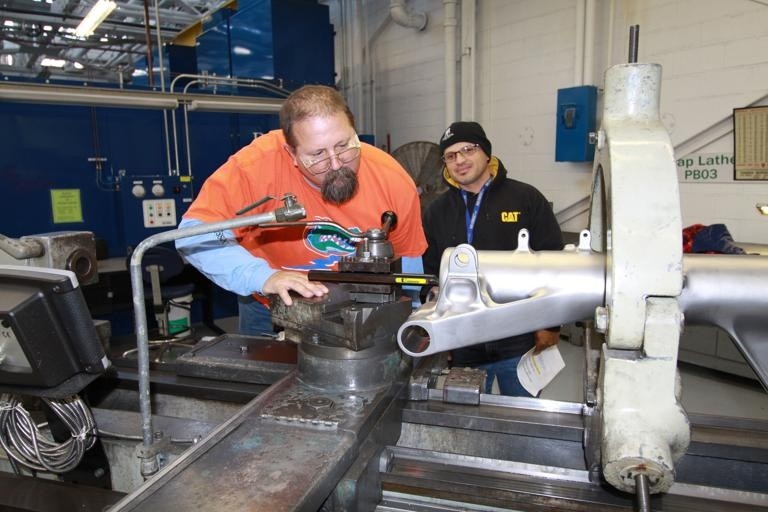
[420,117,566,400]
[172,81,429,335]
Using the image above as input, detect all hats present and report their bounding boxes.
[439,121,491,162]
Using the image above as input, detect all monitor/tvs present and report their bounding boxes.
[0,264,110,387]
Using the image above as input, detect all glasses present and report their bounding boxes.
[298,141,361,176]
[441,144,479,163]
